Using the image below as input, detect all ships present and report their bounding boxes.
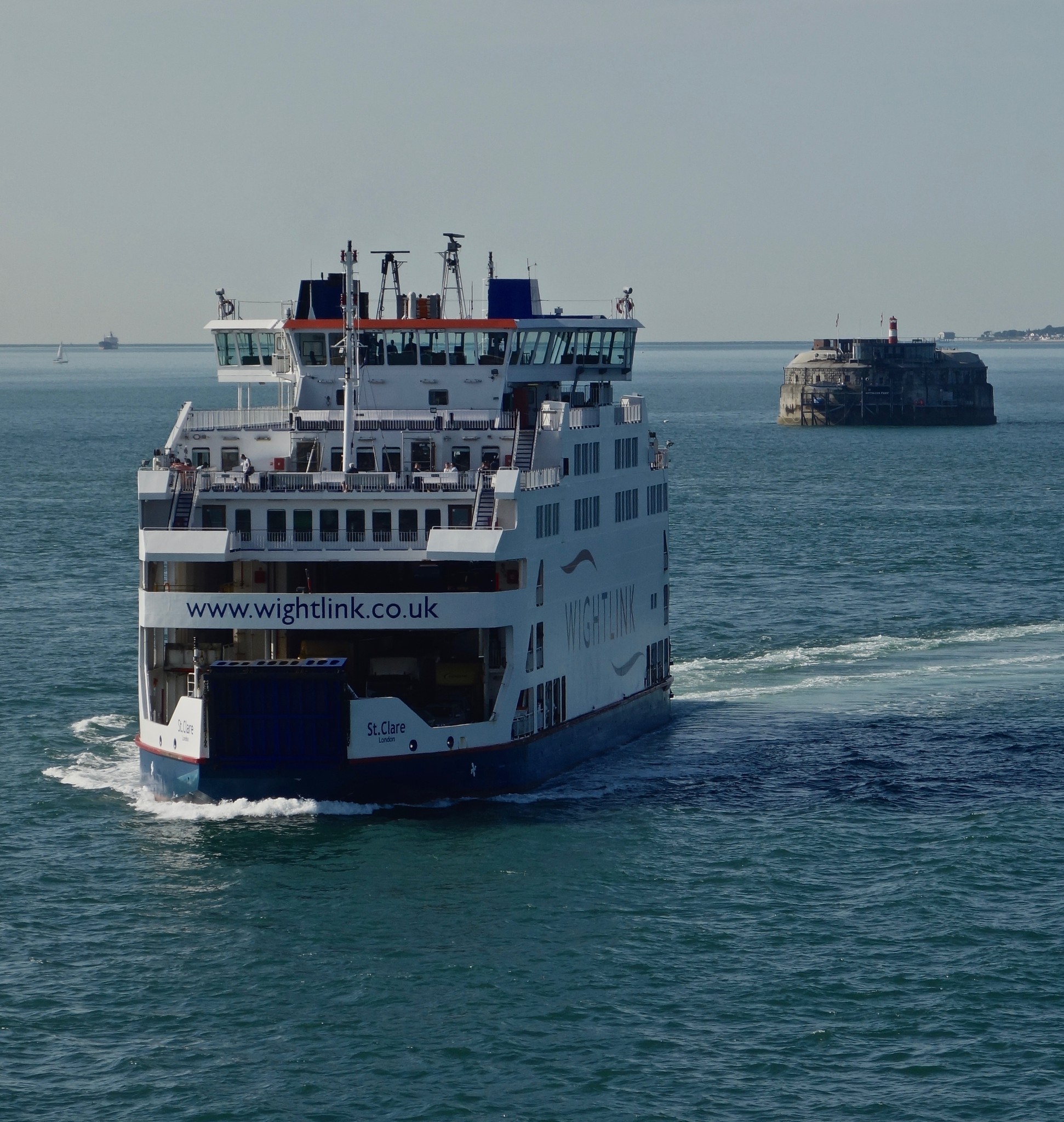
[127,237,679,814]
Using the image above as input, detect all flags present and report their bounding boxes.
[835,313,839,327]
[880,312,883,327]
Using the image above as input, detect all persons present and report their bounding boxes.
[233,477,240,492]
[478,461,490,481]
[387,340,398,355]
[183,457,192,467]
[194,461,209,484]
[341,463,360,492]
[443,462,451,473]
[405,337,417,353]
[411,462,427,492]
[448,462,460,489]
[172,456,181,463]
[240,453,252,491]
[378,339,385,365]
[484,460,493,481]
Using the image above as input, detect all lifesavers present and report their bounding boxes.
[616,299,632,314]
[222,300,234,316]
